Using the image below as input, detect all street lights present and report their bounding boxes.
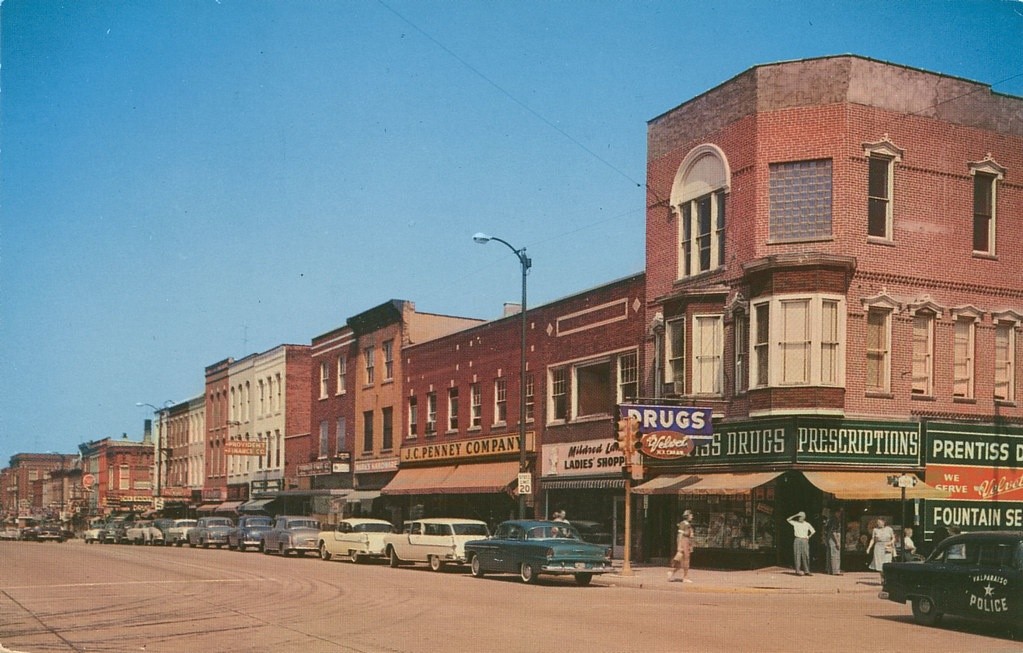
[137,402,162,497]
[473,232,527,517]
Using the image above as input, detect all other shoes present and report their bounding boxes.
[668,572,674,581]
[835,573,843,576]
[806,572,812,576]
[682,577,692,583]
[795,572,804,576]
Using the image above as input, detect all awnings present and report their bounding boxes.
[332,491,382,502]
[802,471,950,500]
[381,465,455,494]
[440,462,529,493]
[631,472,785,496]
[196,499,274,512]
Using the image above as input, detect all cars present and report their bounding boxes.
[464,519,613,587]
[85,520,164,546]
[879,531,1023,628]
[316,518,400,563]
[383,519,492,572]
[259,515,321,557]
[0,526,69,543]
[187,517,237,550]
[163,519,197,547]
[227,515,275,551]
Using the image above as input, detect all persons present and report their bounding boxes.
[552,510,571,539]
[826,508,843,575]
[864,517,896,586]
[904,528,916,561]
[934,525,966,559]
[787,511,815,577]
[668,509,694,583]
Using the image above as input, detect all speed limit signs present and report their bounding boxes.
[518,473,532,494]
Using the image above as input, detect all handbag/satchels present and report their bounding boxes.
[674,551,683,561]
